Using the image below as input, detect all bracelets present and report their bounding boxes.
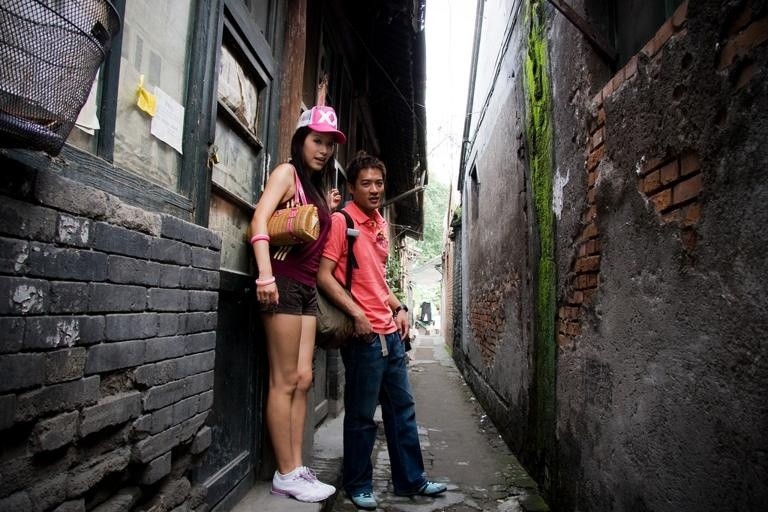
[254,276,277,286]
[395,304,408,315]
[249,235,271,244]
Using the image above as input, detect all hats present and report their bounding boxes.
[294,106,346,145]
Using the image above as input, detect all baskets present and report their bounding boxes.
[1,0,121,157]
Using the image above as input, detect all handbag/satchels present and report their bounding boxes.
[248,166,321,245]
[314,287,353,349]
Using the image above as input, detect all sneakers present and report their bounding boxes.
[351,493,378,509]
[402,481,447,496]
[270,466,336,502]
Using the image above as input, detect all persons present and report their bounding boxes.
[249,104,347,504]
[320,154,448,509]
